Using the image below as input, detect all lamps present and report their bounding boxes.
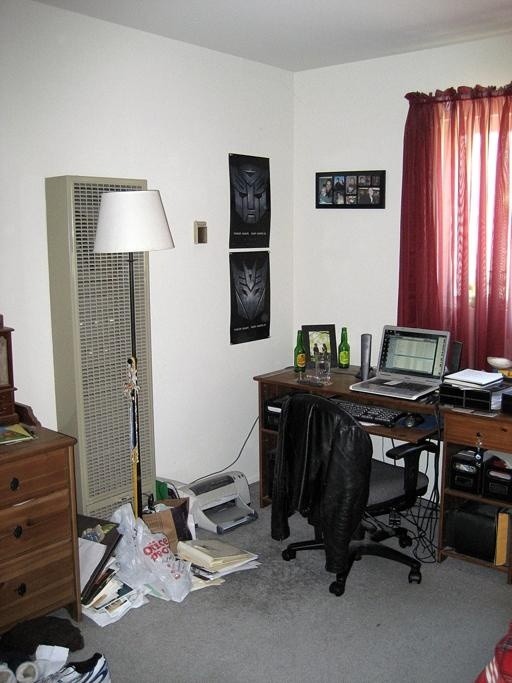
[93,190,177,517]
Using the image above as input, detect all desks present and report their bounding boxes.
[252,361,512,581]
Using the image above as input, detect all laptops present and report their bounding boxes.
[348,324,451,401]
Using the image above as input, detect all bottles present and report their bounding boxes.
[294,329,306,372]
[338,326,351,368]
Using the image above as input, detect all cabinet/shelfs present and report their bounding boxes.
[1,423,84,633]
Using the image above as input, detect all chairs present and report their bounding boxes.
[275,393,439,596]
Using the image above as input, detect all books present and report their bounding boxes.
[444,368,504,393]
[76,511,262,629]
[1,424,32,445]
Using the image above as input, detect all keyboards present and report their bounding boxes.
[326,394,404,428]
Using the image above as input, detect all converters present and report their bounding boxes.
[389,510,401,526]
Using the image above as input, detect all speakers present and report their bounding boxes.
[361,334,372,380]
[451,341,463,372]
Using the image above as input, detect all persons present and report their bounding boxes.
[316,172,382,205]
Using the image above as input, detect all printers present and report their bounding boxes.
[176,471,258,535]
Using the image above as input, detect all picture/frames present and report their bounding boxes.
[301,323,339,369]
[314,169,387,208]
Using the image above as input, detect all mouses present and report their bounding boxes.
[404,413,426,429]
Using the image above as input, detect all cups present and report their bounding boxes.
[298,355,332,385]
